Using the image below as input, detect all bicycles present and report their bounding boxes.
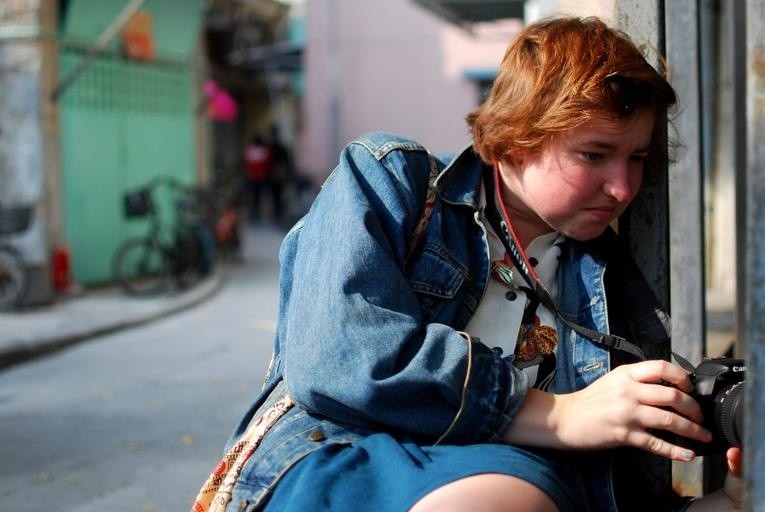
[112,174,217,298]
[0,204,36,310]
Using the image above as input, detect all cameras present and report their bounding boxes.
[658,357,744,457]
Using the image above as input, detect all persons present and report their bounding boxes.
[266,122,295,219]
[245,131,268,217]
[165,199,215,285]
[192,16,743,512]
